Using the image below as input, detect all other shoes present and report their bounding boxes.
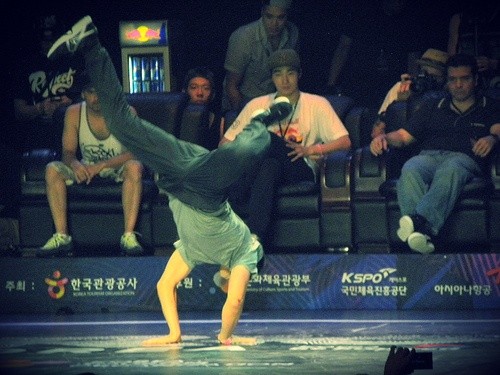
[408,229,435,254]
[396,214,419,242]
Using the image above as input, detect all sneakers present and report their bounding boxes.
[47,15,98,63]
[37,232,73,255]
[120,232,146,255]
[251,100,293,128]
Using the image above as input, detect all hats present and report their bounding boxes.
[416,48,449,71]
[270,49,301,69]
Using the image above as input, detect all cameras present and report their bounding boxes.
[405,69,437,95]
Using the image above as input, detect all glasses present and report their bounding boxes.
[447,75,474,84]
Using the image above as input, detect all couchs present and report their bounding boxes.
[19,92,500,254]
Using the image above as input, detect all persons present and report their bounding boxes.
[223,0,300,130]
[0,0,500,345]
[36,70,145,255]
[45,14,292,345]
[183,65,227,151]
[216,50,353,268]
[369,47,448,139]
[368,53,500,254]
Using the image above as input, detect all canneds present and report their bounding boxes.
[132,55,164,94]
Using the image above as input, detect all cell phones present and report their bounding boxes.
[412,351,433,371]
